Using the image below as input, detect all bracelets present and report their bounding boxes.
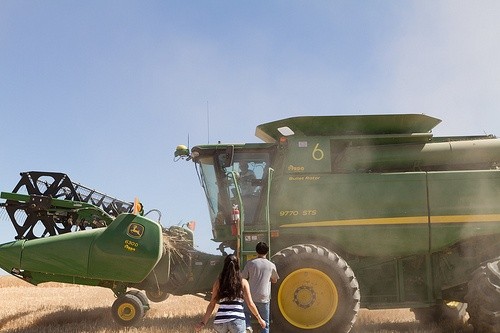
[200,321,205,326]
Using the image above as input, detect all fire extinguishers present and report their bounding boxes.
[230,204,240,236]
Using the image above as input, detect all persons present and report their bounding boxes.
[242,242,277,333]
[231,162,256,196]
[195,254,266,333]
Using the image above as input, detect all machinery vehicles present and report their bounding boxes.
[0,112,500,333]
